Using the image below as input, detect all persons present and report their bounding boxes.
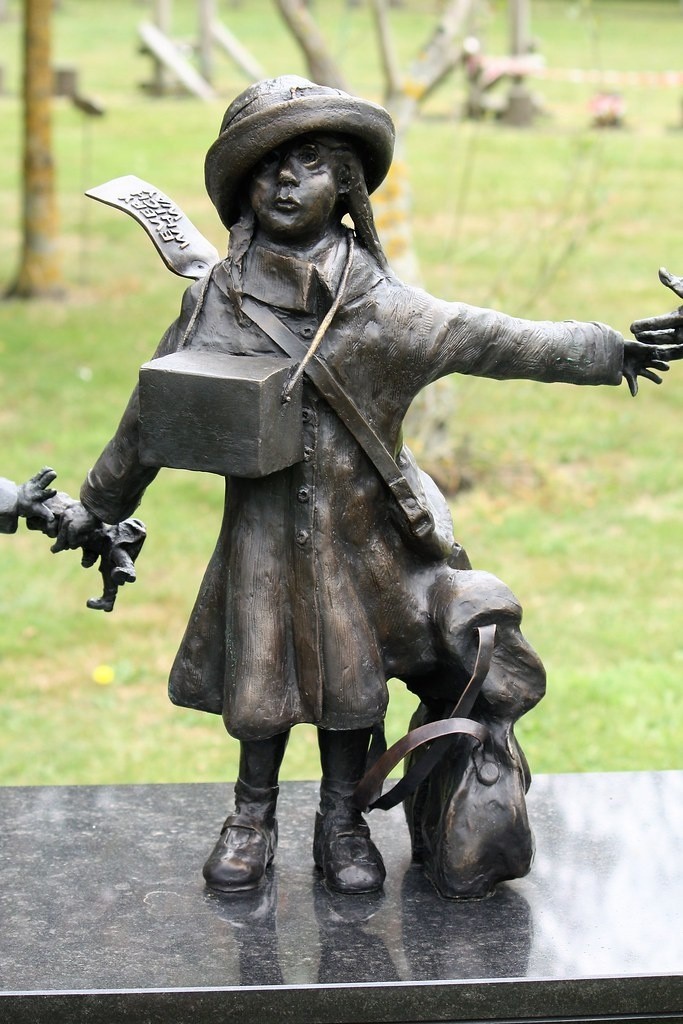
[0,73,683,894]
